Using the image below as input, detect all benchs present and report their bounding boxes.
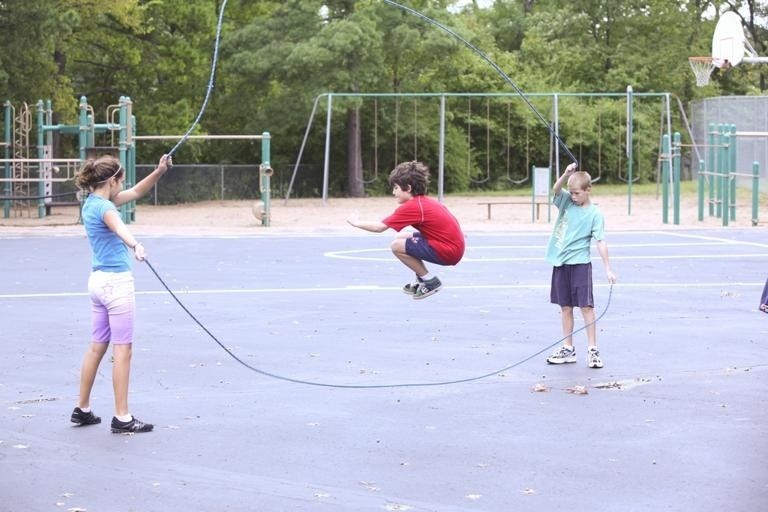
[45,202,81,215]
[477,201,553,220]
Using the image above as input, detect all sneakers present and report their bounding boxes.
[546,346,577,363]
[588,346,603,368]
[71,407,101,423]
[111,416,153,433]
[401,276,442,300]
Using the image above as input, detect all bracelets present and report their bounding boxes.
[132,242,138,249]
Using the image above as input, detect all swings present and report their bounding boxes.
[467,97,489,184]
[354,97,378,183]
[579,100,601,183]
[618,99,641,182]
[506,101,530,183]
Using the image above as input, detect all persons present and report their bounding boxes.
[70,153,174,436]
[543,162,617,369]
[344,160,466,301]
